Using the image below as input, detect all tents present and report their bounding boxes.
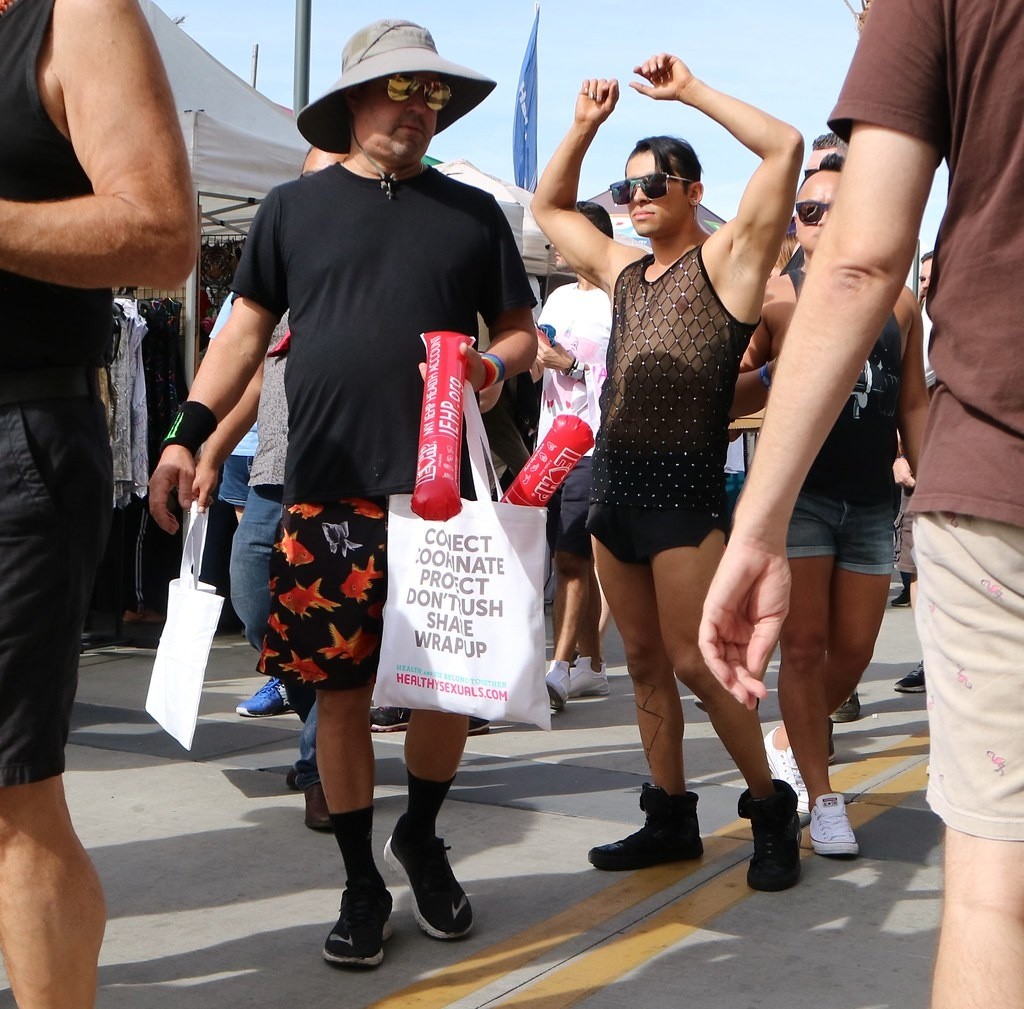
[136,2,728,402]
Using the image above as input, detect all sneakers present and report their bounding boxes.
[762,726,810,828]
[589,783,704,871]
[324,876,394,967]
[811,792,859,856]
[829,688,860,722]
[545,658,572,709]
[383,812,474,940]
[828,717,836,764]
[894,658,926,693]
[236,673,296,717]
[467,714,488,736]
[569,655,610,698]
[368,705,410,730]
[891,585,911,607]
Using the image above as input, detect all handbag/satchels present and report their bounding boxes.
[371,375,551,732]
[145,502,225,752]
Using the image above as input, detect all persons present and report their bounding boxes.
[0,0,200,1009]
[187,127,944,857]
[533,53,805,891]
[149,21,546,968]
[694,0,1023,1009]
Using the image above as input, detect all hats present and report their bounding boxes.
[296,18,497,153]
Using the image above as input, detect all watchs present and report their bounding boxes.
[566,358,584,380]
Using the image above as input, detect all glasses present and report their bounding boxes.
[384,73,451,111]
[610,173,692,205]
[795,201,830,227]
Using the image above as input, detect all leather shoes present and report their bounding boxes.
[286,767,334,829]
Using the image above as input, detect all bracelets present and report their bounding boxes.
[480,353,506,392]
[764,363,770,388]
[773,356,777,364]
[162,400,217,457]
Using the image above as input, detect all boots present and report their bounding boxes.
[737,777,803,891]
